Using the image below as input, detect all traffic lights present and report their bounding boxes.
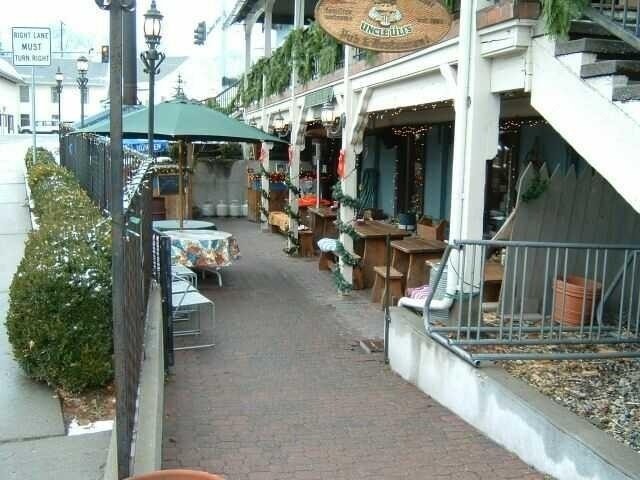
[103,47,108,63]
[197,22,206,40]
[193,26,202,45]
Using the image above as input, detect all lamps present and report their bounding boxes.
[319,93,347,140]
[272,107,293,138]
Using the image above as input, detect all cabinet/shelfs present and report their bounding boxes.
[153,195,189,220]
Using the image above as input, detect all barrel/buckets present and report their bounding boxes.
[424,258,450,325]
[202,200,214,216]
[216,200,227,216]
[553,274,602,326]
[243,200,249,216]
[229,200,241,216]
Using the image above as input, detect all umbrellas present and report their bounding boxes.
[65,93,289,229]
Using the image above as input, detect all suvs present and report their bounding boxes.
[20,118,62,135]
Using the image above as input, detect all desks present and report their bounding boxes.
[164,229,242,287]
[153,219,217,231]
[246,185,509,314]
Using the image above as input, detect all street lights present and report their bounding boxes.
[75,56,89,128]
[142,0,162,160]
[59,21,64,58]
[55,66,65,134]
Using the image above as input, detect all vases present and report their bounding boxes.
[551,274,605,327]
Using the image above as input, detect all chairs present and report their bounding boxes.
[171,261,218,350]
